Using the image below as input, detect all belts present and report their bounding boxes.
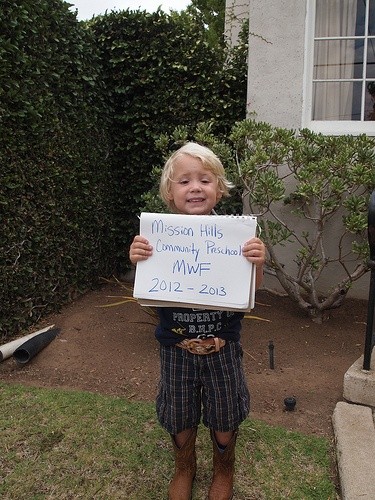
[174,337,227,355]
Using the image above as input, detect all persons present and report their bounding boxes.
[127,142,265,499]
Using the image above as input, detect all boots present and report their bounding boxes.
[168,426,197,500]
[208,428,237,500]
